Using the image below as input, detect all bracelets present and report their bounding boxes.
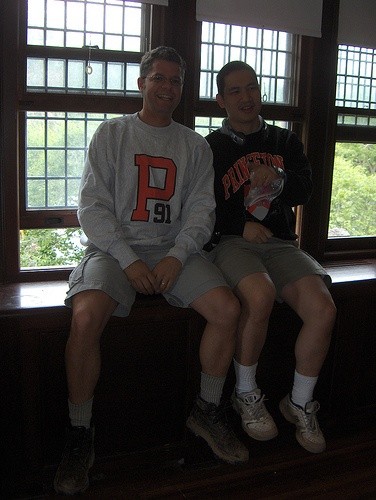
[270,165,286,177]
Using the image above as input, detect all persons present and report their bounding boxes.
[54,46,249,495]
[198,61,336,453]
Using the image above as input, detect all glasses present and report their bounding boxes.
[143,73,185,90]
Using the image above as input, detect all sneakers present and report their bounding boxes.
[231,387,278,441]
[186,394,250,465]
[55,425,98,498]
[279,393,326,454]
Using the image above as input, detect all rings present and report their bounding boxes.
[162,282,166,288]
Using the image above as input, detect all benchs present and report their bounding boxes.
[0,257,376,471]
